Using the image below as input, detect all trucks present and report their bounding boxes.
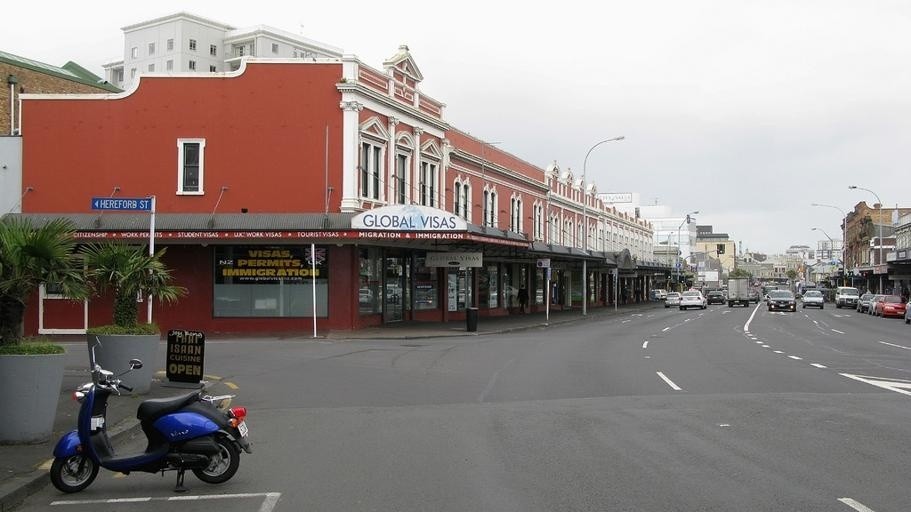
[724,276,751,310]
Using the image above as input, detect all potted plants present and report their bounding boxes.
[1,214,88,445]
[78,239,190,394]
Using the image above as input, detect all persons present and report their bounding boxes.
[621,285,643,305]
[517,285,530,315]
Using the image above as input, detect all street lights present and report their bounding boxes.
[810,227,833,261]
[810,202,847,286]
[847,183,885,295]
[580,135,626,316]
[666,211,701,286]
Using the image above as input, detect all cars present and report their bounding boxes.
[904,299,911,324]
[753,281,770,288]
[356,281,544,307]
[652,286,907,319]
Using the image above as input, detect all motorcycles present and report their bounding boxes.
[45,334,255,494]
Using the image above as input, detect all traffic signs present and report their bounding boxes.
[90,197,152,211]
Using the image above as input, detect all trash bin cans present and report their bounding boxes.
[465,304,479,332]
